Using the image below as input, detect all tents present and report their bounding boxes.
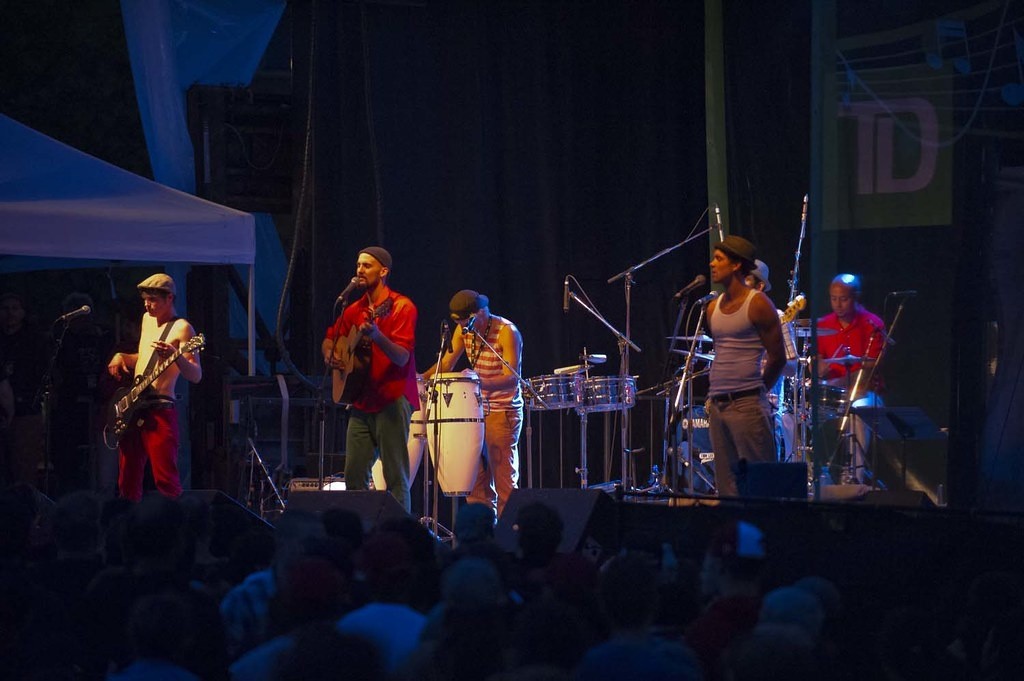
[0,113,289,378]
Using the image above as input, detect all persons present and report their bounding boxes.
[746,259,798,462]
[422,289,523,520]
[109,274,202,502]
[0,275,1024,681]
[706,236,786,496]
[806,273,889,482]
[322,246,420,514]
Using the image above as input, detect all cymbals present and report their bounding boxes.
[822,356,874,363]
[666,334,713,343]
[794,327,838,336]
[672,347,714,362]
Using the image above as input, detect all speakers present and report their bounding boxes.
[852,406,950,505]
[491,485,631,559]
[268,487,445,567]
[732,462,808,502]
[246,397,319,483]
[164,489,272,560]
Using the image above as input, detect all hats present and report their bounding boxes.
[358,247,392,271]
[449,290,488,321]
[749,259,771,292]
[137,273,176,298]
[712,235,758,271]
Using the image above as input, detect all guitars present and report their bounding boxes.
[106,333,206,440]
[332,296,394,405]
[782,291,807,324]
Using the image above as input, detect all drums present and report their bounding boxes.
[371,372,426,492]
[425,372,485,497]
[522,374,584,408]
[781,375,850,417]
[665,400,800,492]
[577,377,636,410]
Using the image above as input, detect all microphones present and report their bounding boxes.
[802,195,807,222]
[674,275,704,297]
[869,320,899,345]
[696,291,718,304]
[889,291,916,297]
[715,208,725,242]
[462,316,476,334]
[566,281,569,314]
[337,277,360,301]
[61,305,91,319]
[443,319,452,353]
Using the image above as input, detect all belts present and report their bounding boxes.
[708,386,764,403]
